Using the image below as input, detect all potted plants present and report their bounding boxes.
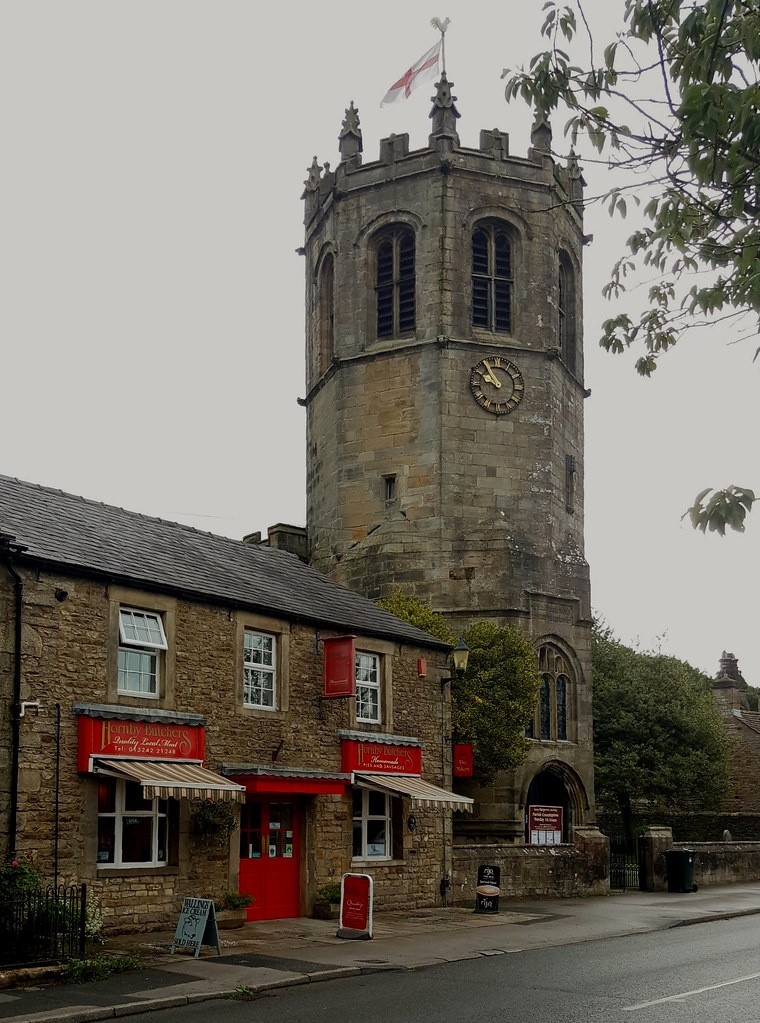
[312,882,341,919]
[214,893,255,928]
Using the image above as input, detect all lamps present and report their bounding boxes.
[440,635,472,695]
[408,814,417,832]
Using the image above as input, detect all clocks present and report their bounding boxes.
[471,356,526,415]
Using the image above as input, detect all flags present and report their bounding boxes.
[379,38,442,108]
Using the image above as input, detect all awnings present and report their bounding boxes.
[88,754,474,815]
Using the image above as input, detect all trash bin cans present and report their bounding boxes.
[661,849,699,893]
